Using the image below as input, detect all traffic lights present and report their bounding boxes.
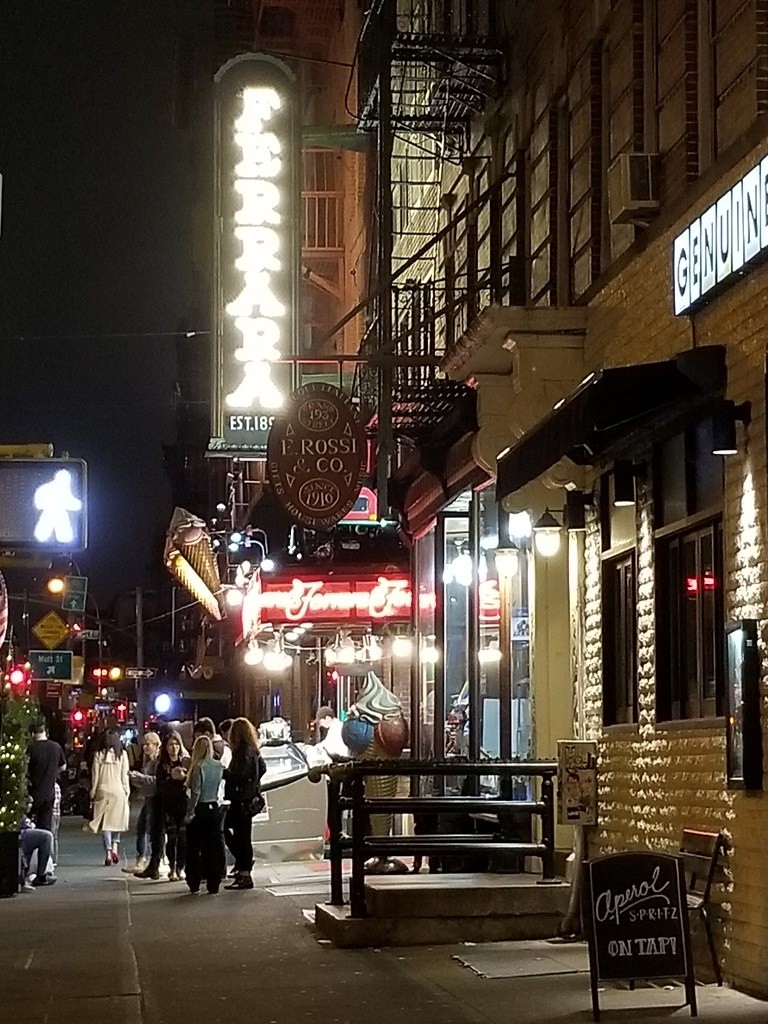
[0,459,88,551]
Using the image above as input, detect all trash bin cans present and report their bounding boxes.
[0,828,22,899]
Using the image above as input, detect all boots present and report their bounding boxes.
[134,857,160,880]
[122,857,144,872]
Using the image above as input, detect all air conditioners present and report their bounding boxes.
[604,152,658,221]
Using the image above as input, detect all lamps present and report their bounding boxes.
[533,489,594,557]
[613,452,647,508]
[454,529,520,586]
[715,399,753,455]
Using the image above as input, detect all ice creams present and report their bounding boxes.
[341,671,408,836]
[162,507,226,621]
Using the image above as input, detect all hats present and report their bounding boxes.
[311,706,334,725]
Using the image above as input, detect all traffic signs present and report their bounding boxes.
[61,576,89,612]
[126,667,157,679]
[29,650,73,682]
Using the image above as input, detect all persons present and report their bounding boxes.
[29,725,66,867]
[220,718,266,890]
[182,717,227,895]
[16,823,58,892]
[84,729,130,866]
[121,726,190,884]
[313,707,350,758]
[52,780,62,862]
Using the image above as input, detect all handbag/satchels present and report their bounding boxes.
[83,799,94,821]
[240,793,265,819]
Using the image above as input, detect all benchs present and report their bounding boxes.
[628,829,722,990]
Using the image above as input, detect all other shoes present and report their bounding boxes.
[112,852,118,864]
[224,875,253,889]
[178,868,186,879]
[227,873,239,877]
[31,874,57,886]
[221,874,226,881]
[192,890,200,894]
[201,877,207,883]
[105,858,111,865]
[169,868,179,881]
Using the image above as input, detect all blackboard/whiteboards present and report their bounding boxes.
[587,854,687,992]
